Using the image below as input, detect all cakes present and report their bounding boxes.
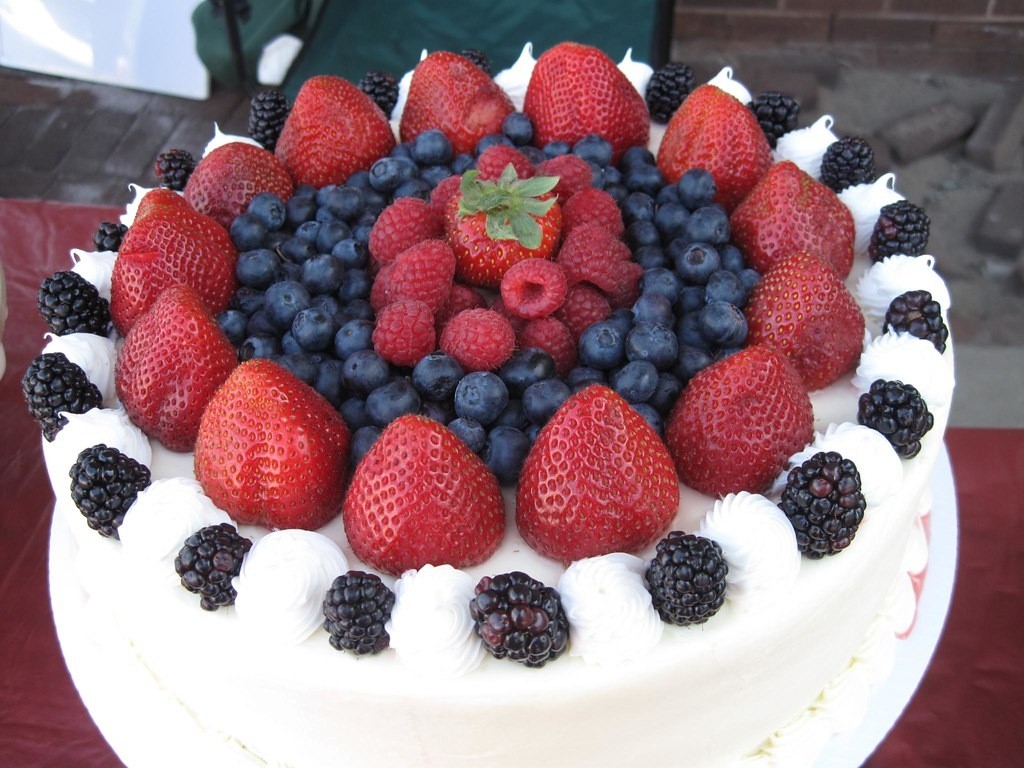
[23,40,956,767]
